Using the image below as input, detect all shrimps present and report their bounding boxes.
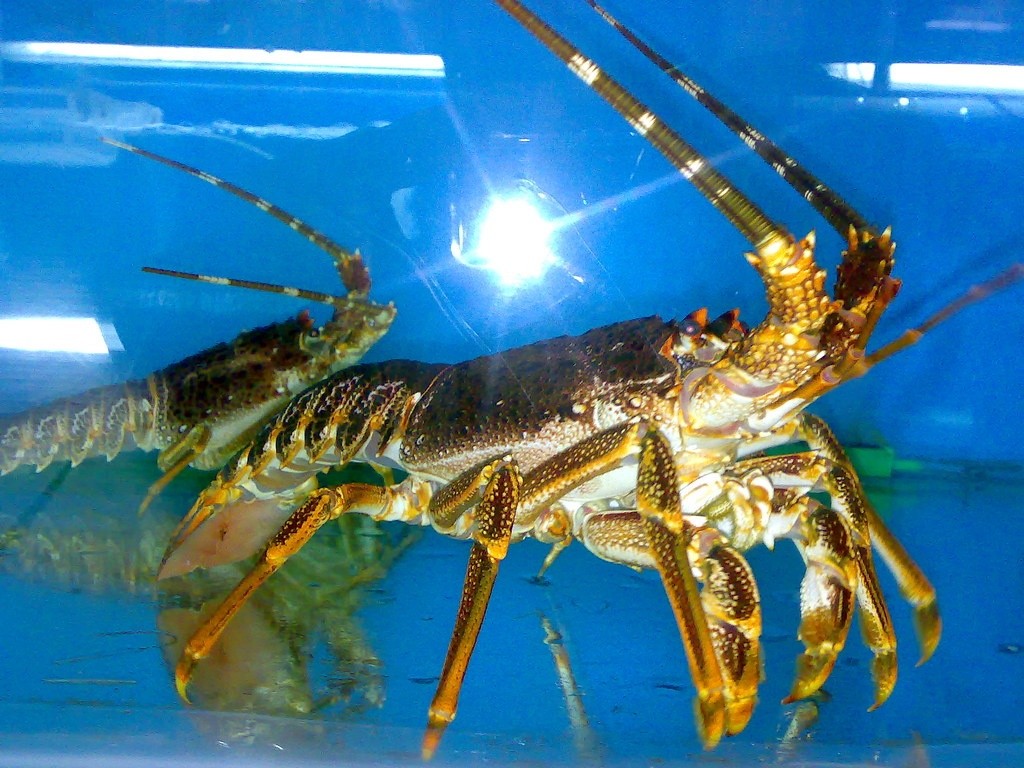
[0,0,1024,768]
